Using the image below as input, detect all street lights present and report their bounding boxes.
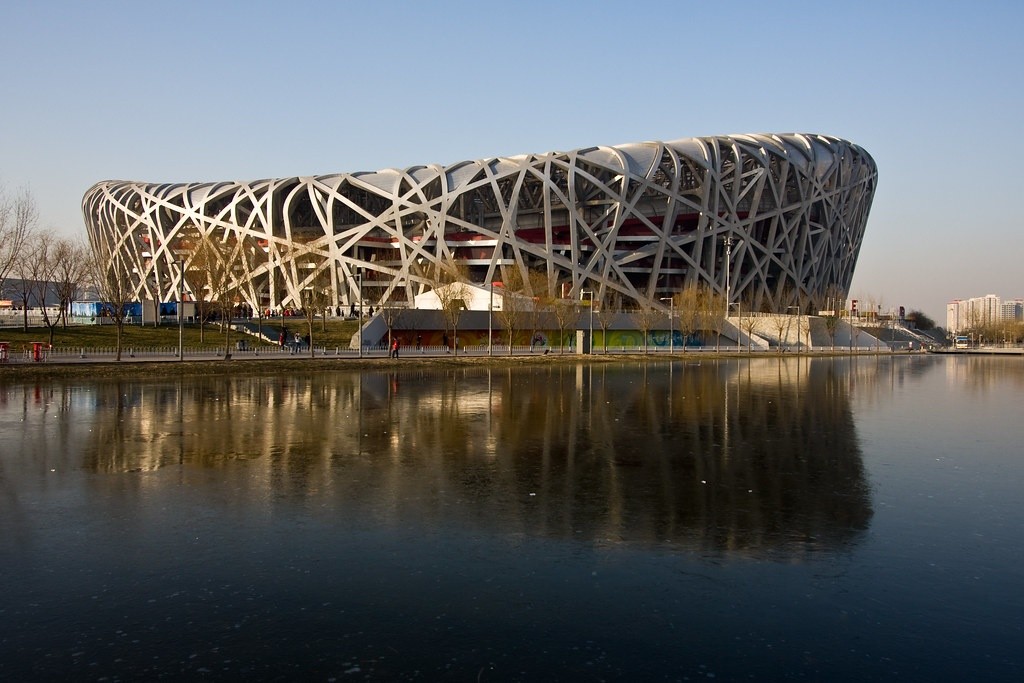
[348,273,362,358]
[787,305,800,354]
[968,333,974,349]
[579,291,593,355]
[167,259,184,361]
[479,283,493,356]
[659,297,674,355]
[729,302,741,352]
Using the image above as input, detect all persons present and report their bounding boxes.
[416,335,423,349]
[294,332,302,352]
[234,305,252,318]
[392,337,400,360]
[443,333,448,347]
[305,333,310,352]
[920,339,925,349]
[909,340,912,352]
[264,307,303,320]
[126,306,135,322]
[456,335,459,350]
[280,326,288,351]
[369,306,374,317]
[336,306,340,316]
[96,306,112,317]
[349,303,355,317]
